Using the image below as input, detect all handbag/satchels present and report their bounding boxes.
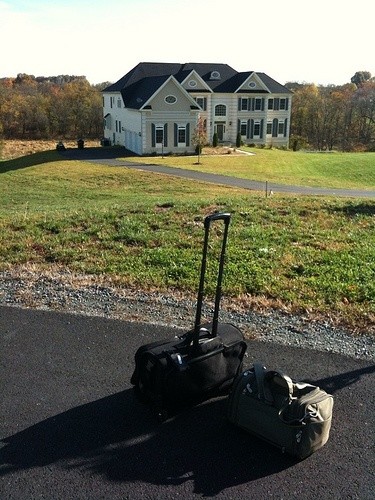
[231,364,336,458]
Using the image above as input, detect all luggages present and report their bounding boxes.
[130,213,248,419]
